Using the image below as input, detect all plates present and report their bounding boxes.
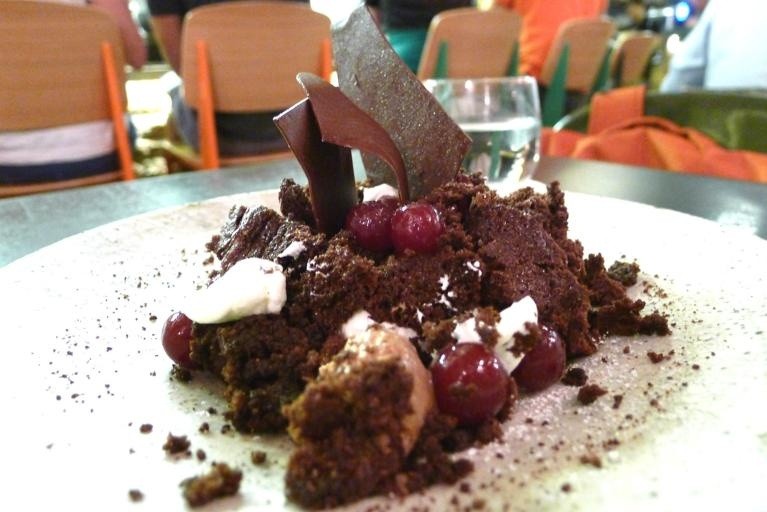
[0,183,767,511]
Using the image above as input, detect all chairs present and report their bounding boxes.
[417,5,524,80]
[610,28,656,88]
[159,2,332,171]
[539,16,612,134]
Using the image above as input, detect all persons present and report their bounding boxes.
[146,0,312,160]
[365,0,477,78]
[494,0,610,114]
[657,1,767,90]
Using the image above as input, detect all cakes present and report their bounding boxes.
[161,6,673,511]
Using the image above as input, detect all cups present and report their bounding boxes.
[421,75,544,184]
